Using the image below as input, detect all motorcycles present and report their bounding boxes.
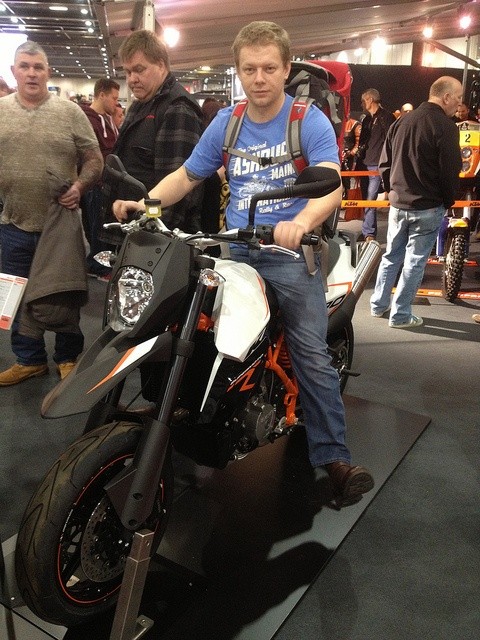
[14,154,381,625]
[442,120,480,301]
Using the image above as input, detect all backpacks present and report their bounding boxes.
[221,61,354,246]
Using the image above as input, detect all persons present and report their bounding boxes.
[93,31,202,235]
[112,20,374,507]
[357,88,397,242]
[0,40,105,386]
[370,76,463,329]
[339,108,363,199]
[401,103,413,115]
[78,78,120,282]
[452,103,480,241]
[0,78,17,98]
[112,103,124,133]
[200,97,227,128]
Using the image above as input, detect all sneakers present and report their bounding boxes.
[0,363,49,385]
[395,315,423,328]
[328,461,375,509]
